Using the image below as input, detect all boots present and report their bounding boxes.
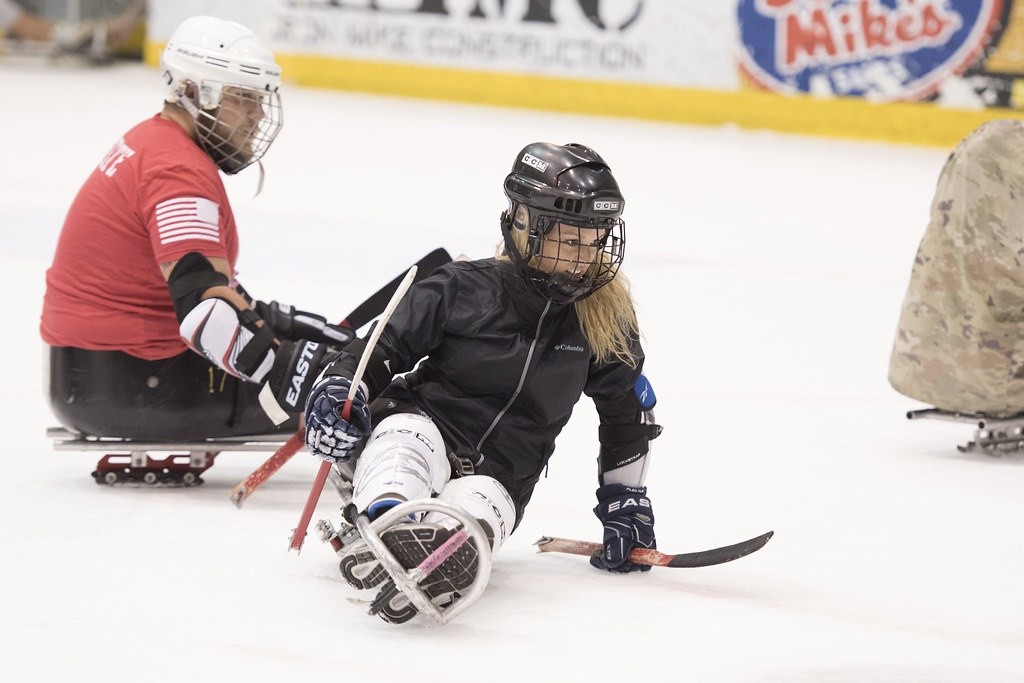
[333,412,455,590]
[370,475,516,646]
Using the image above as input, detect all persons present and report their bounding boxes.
[39,16,359,439]
[304,140,664,626]
[886,117,1024,421]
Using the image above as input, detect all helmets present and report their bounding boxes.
[500,142,626,316]
[159,18,288,174]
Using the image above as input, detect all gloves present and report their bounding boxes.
[590,484,658,573]
[259,300,356,344]
[263,344,340,419]
[304,354,371,462]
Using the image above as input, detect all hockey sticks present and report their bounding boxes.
[291,267,420,558]
[536,534,778,569]
[338,246,454,330]
[233,427,313,510]
